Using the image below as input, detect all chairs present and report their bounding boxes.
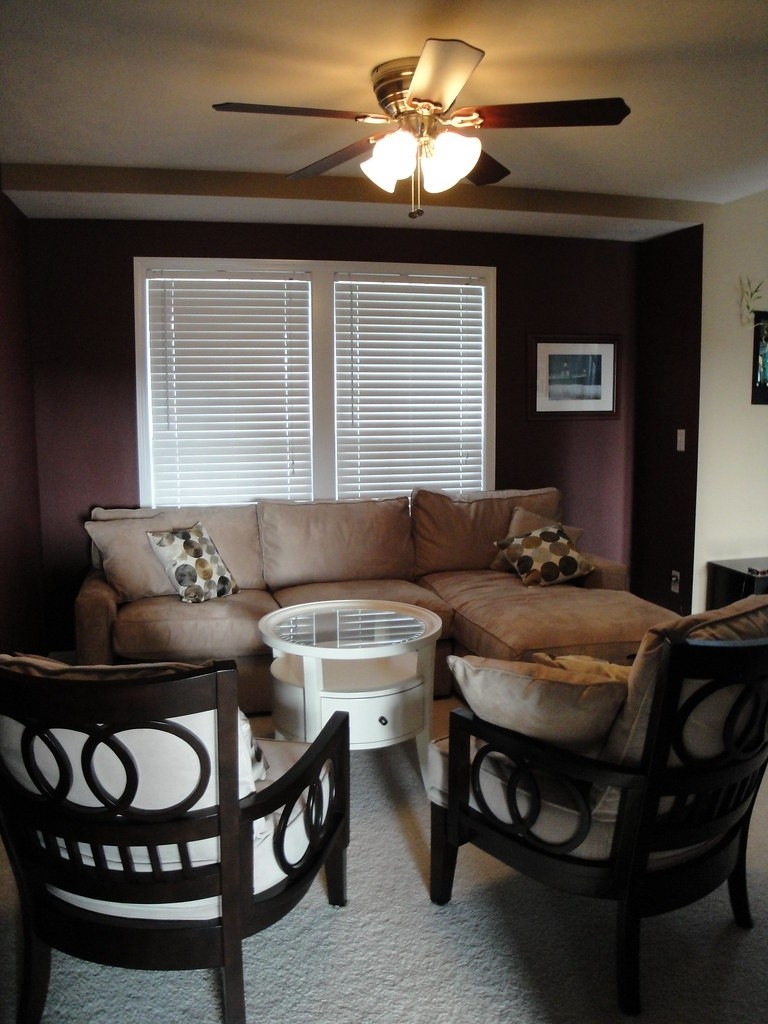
[429,592,768,1017]
[0,651,350,1023]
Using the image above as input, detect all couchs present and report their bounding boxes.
[75,487,684,716]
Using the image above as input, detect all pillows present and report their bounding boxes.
[490,504,585,573]
[85,512,177,600]
[493,521,595,588]
[445,653,632,746]
[148,521,241,604]
[1,652,271,864]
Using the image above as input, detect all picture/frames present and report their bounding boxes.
[526,334,620,422]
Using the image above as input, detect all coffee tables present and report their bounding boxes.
[259,599,443,790]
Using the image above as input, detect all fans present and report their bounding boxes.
[212,37,631,219]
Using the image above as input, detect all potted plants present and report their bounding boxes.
[744,277,768,386]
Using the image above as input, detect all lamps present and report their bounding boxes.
[359,117,483,195]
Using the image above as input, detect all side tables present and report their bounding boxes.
[706,558,768,610]
